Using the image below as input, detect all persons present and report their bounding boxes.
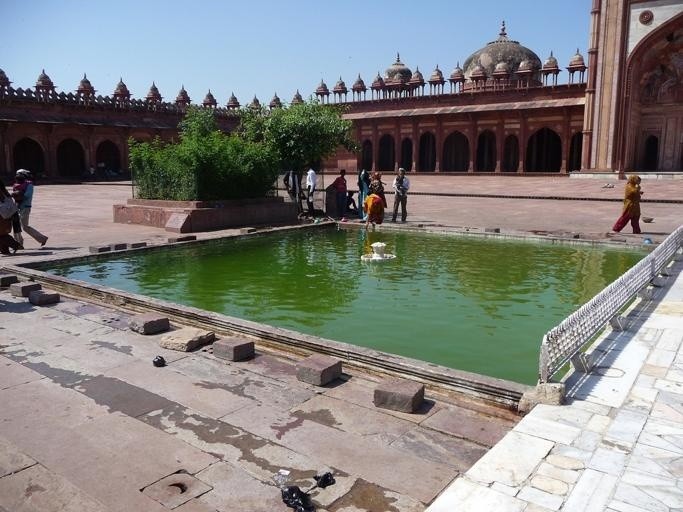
[12,168,48,249]
[327,169,350,222]
[357,165,388,223]
[304,164,316,219]
[611,175,644,234]
[88,159,112,181]
[347,191,359,214]
[391,168,409,223]
[283,168,306,217]
[0,180,20,255]
[13,174,33,208]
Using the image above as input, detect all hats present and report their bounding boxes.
[17,169,30,177]
[399,168,406,172]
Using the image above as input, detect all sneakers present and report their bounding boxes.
[1,237,48,254]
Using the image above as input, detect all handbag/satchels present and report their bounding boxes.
[0,198,18,219]
